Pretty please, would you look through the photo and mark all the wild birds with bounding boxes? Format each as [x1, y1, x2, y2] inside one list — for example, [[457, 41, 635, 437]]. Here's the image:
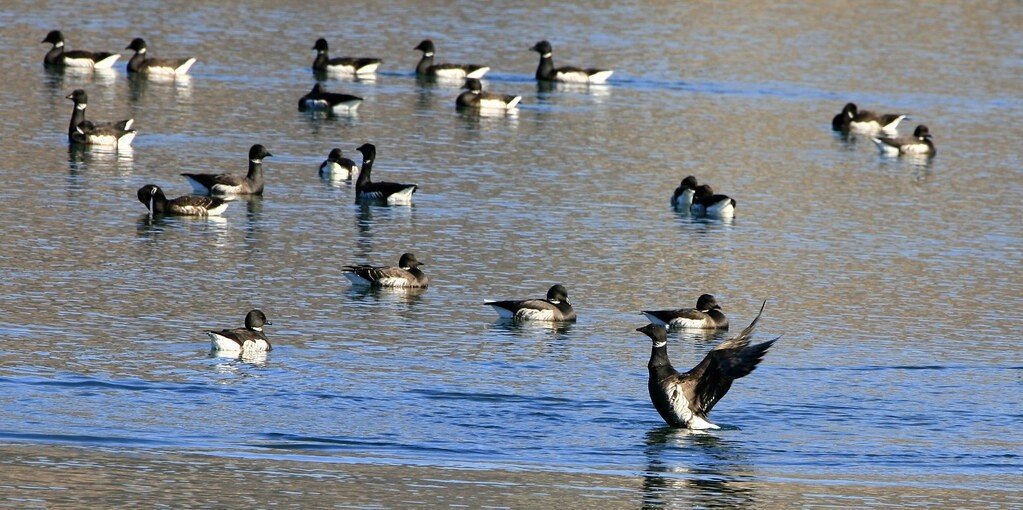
[[638, 294, 730, 330], [124, 38, 196, 75], [636, 300, 786, 430], [319, 172, 358, 188], [65, 90, 137, 145], [41, 31, 121, 69], [341, 253, 429, 289], [832, 102, 937, 154], [137, 185, 229, 216], [413, 41, 523, 109], [639, 428, 757, 510], [203, 310, 272, 351], [484, 284, 578, 322], [356, 143, 420, 202], [180, 144, 273, 195], [298, 38, 385, 113], [319, 147, 358, 174], [670, 175, 736, 217], [529, 41, 613, 84]]
